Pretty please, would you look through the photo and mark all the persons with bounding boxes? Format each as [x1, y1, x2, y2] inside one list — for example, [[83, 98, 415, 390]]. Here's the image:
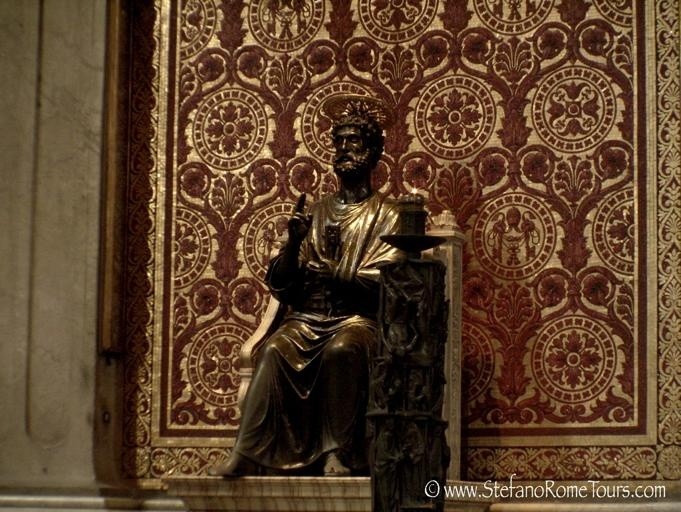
[[209, 104, 402, 477]]
